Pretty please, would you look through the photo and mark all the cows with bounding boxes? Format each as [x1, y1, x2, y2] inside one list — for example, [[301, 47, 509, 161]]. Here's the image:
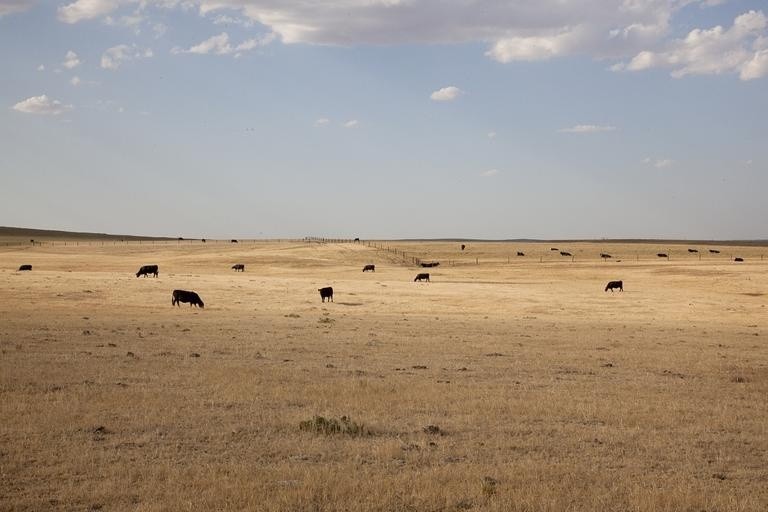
[[172, 289, 205, 309], [734, 256, 744, 261], [413, 273, 431, 283], [317, 286, 335, 303], [19, 264, 33, 271], [604, 280, 624, 292], [599, 252, 612, 259], [134, 264, 160, 278], [657, 252, 667, 257], [546, 246, 572, 258], [231, 264, 245, 273], [361, 264, 376, 273], [688, 247, 720, 254]]
